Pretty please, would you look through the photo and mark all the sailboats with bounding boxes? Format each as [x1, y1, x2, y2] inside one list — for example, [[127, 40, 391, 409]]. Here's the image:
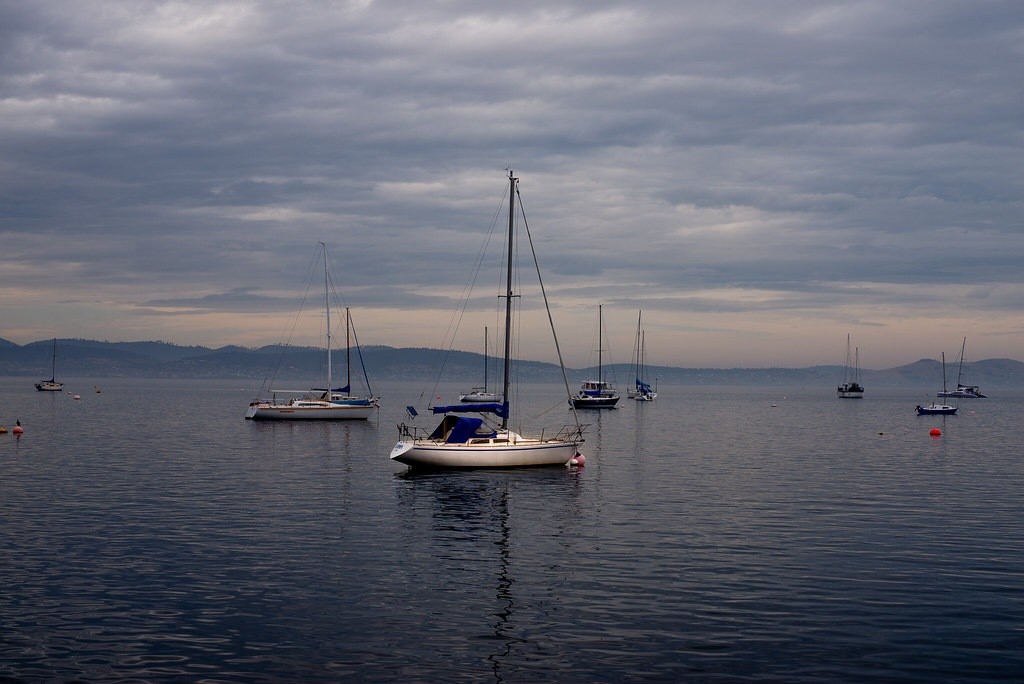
[[626, 309, 659, 401], [568, 302, 622, 411], [243, 240, 381, 422], [836, 333, 865, 399], [912, 351, 959, 415], [33, 337, 66, 391], [937, 335, 989, 398], [388, 168, 592, 470]]
